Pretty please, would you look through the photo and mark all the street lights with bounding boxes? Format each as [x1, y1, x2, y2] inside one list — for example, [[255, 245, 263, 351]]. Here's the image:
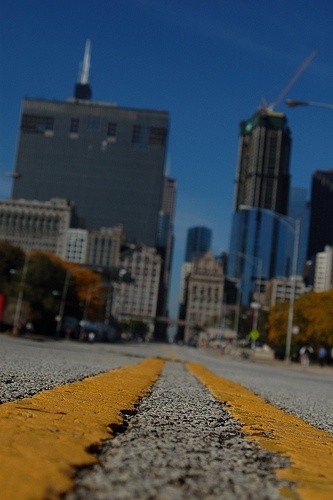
[[238, 204, 302, 360]]
